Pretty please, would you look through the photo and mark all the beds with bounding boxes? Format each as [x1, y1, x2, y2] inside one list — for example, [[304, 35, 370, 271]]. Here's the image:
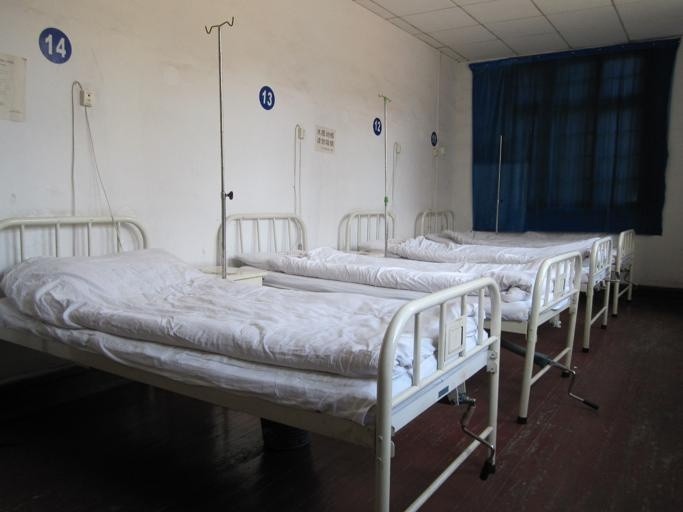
[[3, 207, 501, 510], [211, 210, 637, 426]]
